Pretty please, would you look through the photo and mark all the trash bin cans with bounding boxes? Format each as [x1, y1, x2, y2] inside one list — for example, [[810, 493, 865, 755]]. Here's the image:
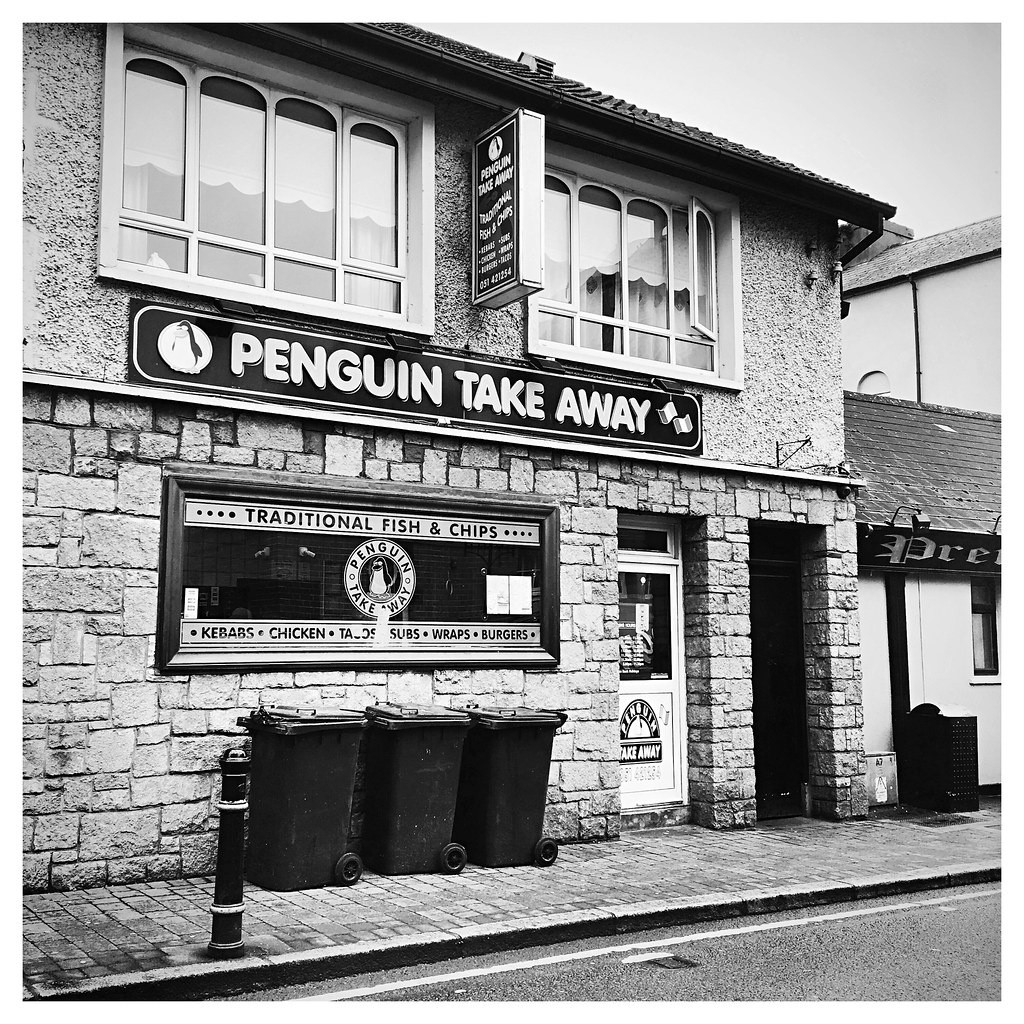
[[237, 706, 360, 893], [358, 702, 467, 875], [461, 706, 568, 868]]
